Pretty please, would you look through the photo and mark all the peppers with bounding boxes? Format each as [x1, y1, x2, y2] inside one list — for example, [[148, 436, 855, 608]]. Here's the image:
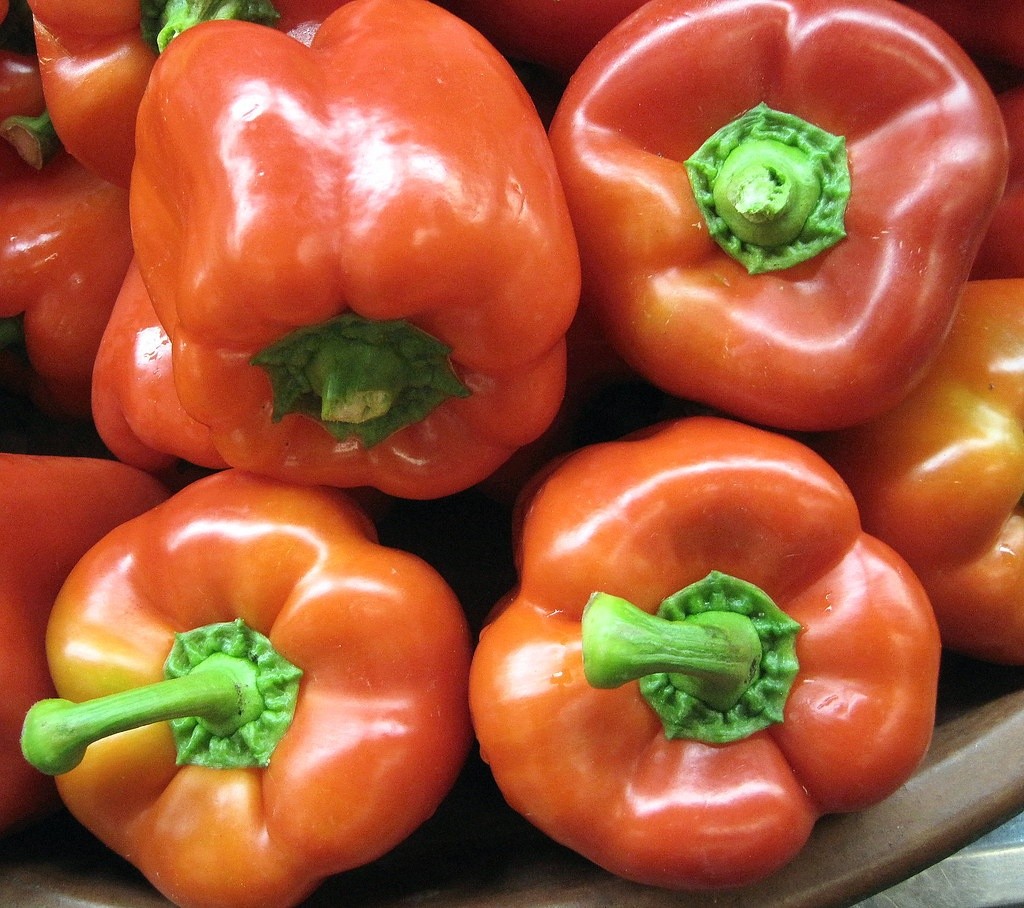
[[0, 0, 1024, 908]]
